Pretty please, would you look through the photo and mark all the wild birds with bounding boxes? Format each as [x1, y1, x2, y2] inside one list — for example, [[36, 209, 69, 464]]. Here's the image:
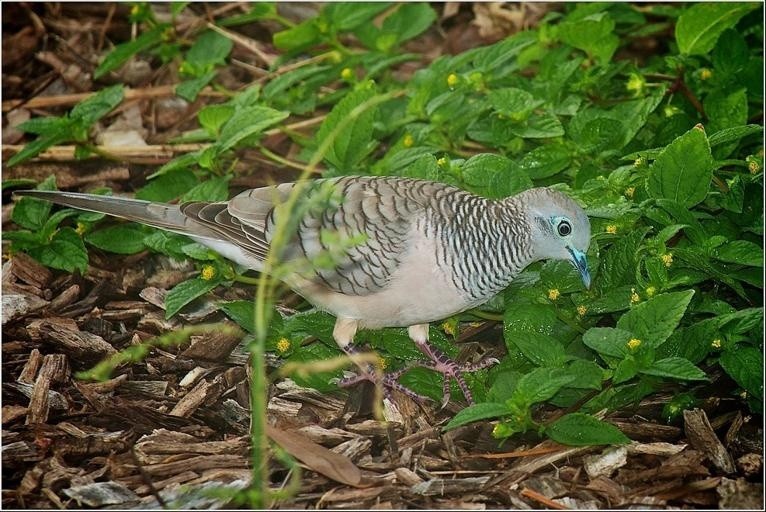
[[10, 175, 590, 410]]
